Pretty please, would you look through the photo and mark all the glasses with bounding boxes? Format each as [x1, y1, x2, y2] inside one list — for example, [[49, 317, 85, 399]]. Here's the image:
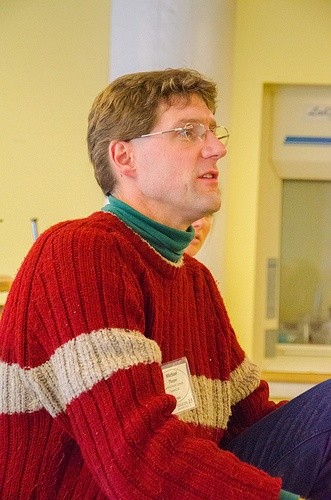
[[202, 215, 215, 223], [126, 123, 230, 147]]
